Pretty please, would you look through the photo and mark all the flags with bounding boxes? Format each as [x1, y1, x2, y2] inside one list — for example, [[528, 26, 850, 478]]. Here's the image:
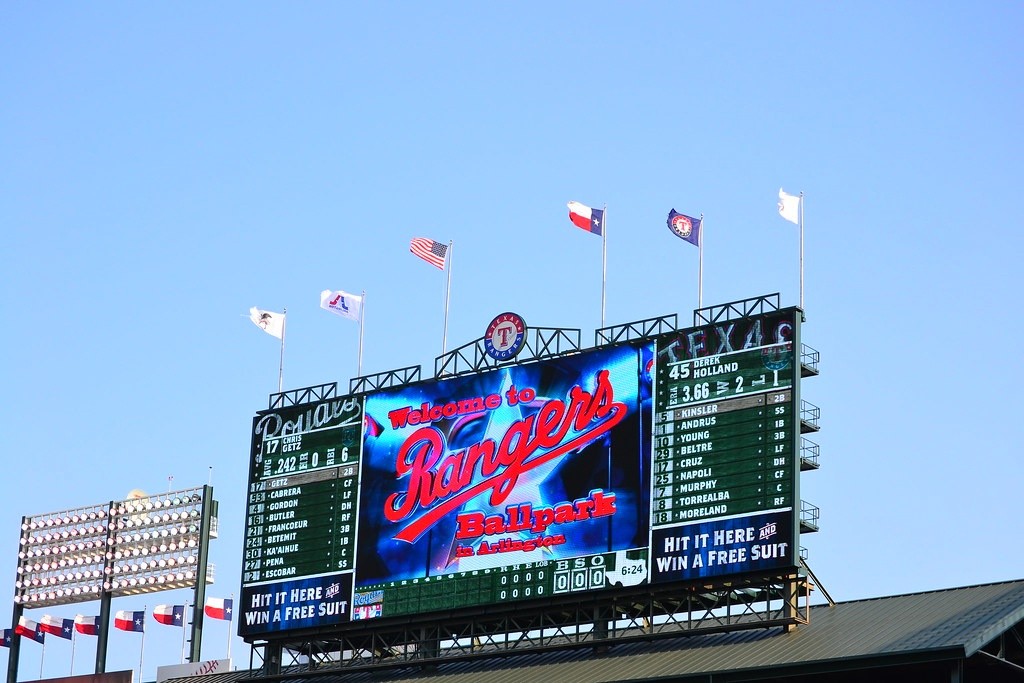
[[410, 237, 448, 271], [778, 187, 800, 225], [74, 614, 100, 635], [114, 610, 145, 633], [320, 289, 361, 322], [0, 628, 12, 648], [566, 199, 603, 236], [249, 307, 283, 339], [153, 604, 184, 627], [40, 614, 74, 640], [666, 208, 701, 247], [204, 597, 232, 620], [15, 616, 44, 644]]
